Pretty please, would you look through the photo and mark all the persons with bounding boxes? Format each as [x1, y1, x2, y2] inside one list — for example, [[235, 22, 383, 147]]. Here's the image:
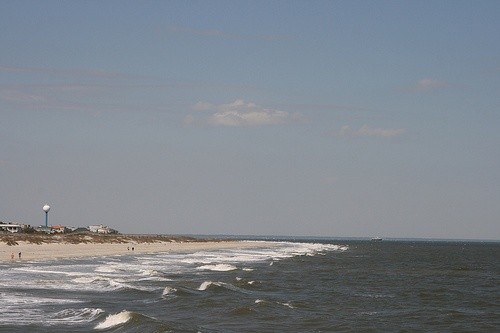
[[11, 253, 15, 260], [132, 246, 135, 251], [127, 246, 130, 251], [19, 252, 22, 259]]
